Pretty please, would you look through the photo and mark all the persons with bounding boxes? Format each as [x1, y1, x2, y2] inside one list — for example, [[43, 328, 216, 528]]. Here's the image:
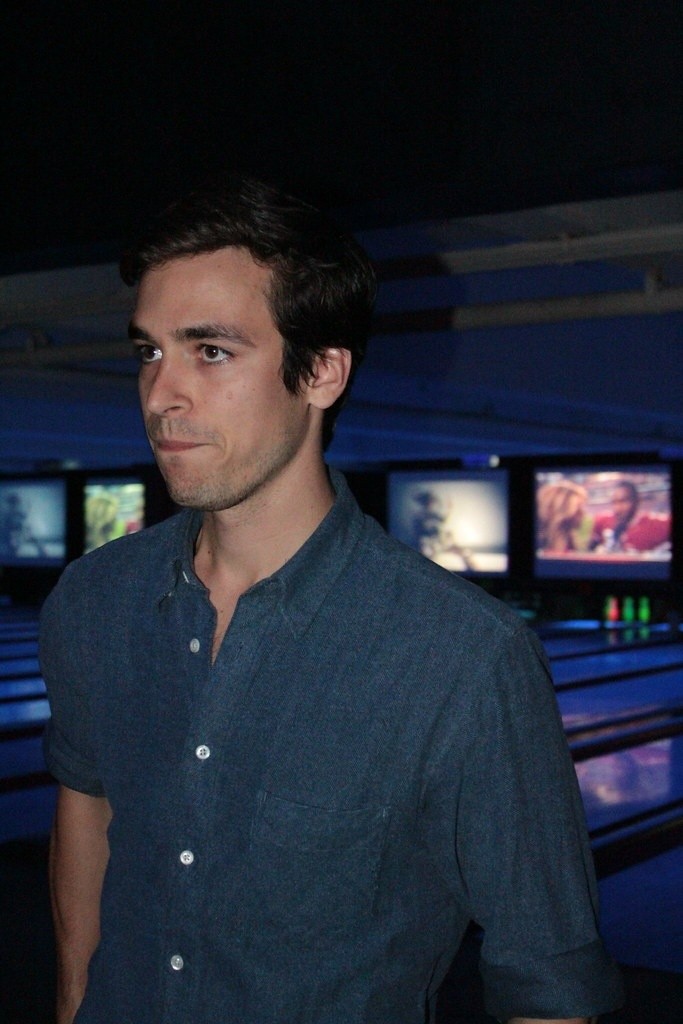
[[536, 475, 591, 559], [41, 178, 603, 1023], [410, 484, 479, 571], [588, 478, 672, 556]]
